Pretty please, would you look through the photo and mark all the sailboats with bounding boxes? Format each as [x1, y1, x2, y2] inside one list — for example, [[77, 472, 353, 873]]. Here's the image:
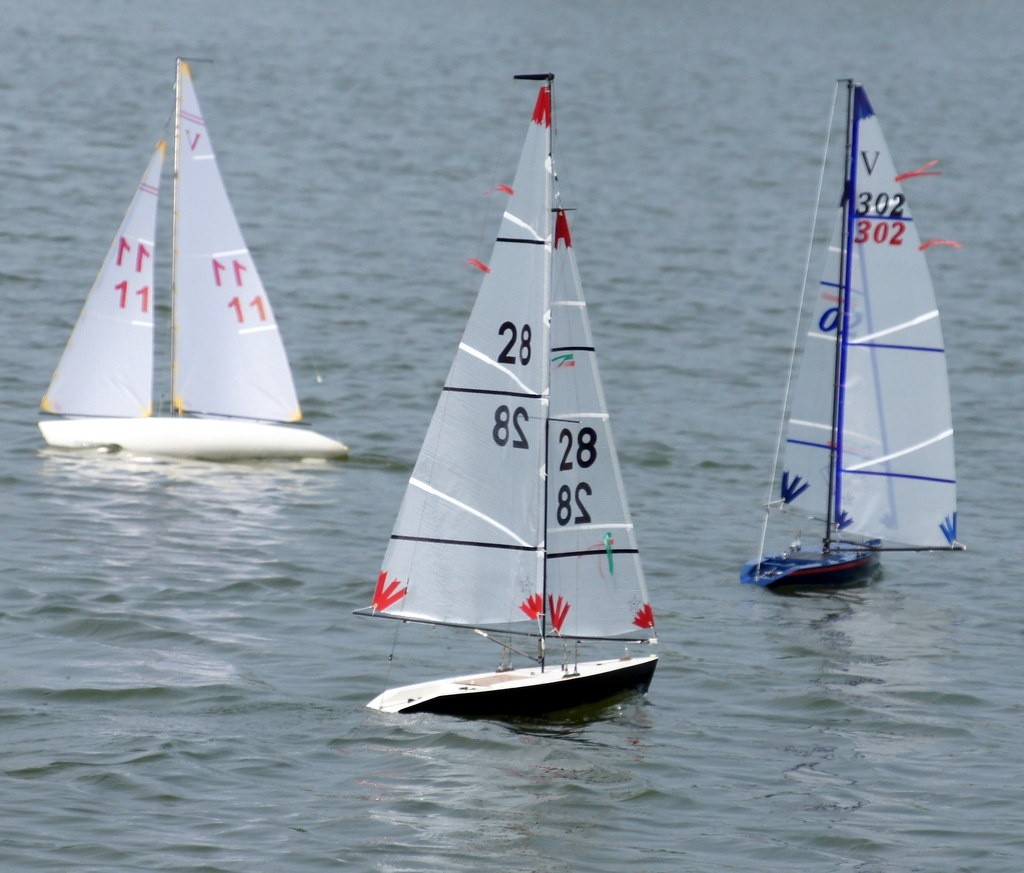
[[738, 80, 968, 591], [351, 69, 659, 718], [36, 53, 349, 462]]
